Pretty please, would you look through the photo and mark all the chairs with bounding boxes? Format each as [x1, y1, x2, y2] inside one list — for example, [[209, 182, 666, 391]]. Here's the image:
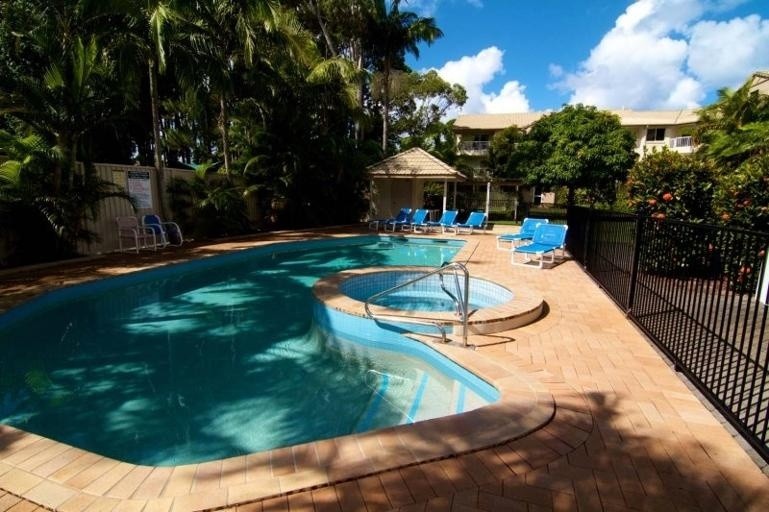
[[497, 218, 549, 252], [115, 215, 157, 253], [511, 223, 568, 268], [368, 208, 486, 235], [141, 215, 183, 251]]
